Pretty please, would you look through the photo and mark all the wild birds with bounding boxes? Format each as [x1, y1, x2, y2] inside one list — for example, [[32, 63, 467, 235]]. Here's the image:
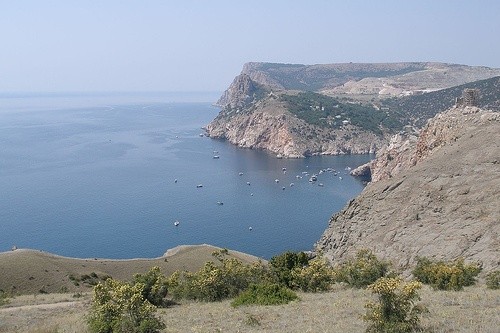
[[174, 220, 180, 226], [239, 172, 244, 176], [197, 184, 203, 188], [217, 202, 223, 205], [212, 155, 219, 159], [249, 225, 252, 230], [275, 165, 351, 190], [246, 182, 251, 186]]
